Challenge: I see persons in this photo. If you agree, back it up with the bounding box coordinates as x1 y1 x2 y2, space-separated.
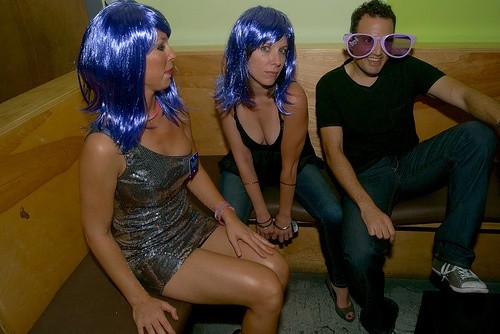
75 1 289 334
212 6 357 323
316 0 500 334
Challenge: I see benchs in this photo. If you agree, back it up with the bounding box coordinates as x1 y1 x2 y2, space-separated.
0 45 500 334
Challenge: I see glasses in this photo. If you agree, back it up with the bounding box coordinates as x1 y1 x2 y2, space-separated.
343 33 416 58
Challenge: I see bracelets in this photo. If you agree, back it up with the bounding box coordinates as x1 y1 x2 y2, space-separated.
280 182 296 187
256 216 273 228
214 200 236 225
243 181 258 185
274 220 291 230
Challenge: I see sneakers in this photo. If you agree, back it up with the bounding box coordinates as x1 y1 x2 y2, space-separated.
431 255 489 294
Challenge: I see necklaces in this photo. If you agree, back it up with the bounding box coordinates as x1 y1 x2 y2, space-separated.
142 101 161 123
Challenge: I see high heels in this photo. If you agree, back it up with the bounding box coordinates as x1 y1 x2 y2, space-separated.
325 272 356 323
